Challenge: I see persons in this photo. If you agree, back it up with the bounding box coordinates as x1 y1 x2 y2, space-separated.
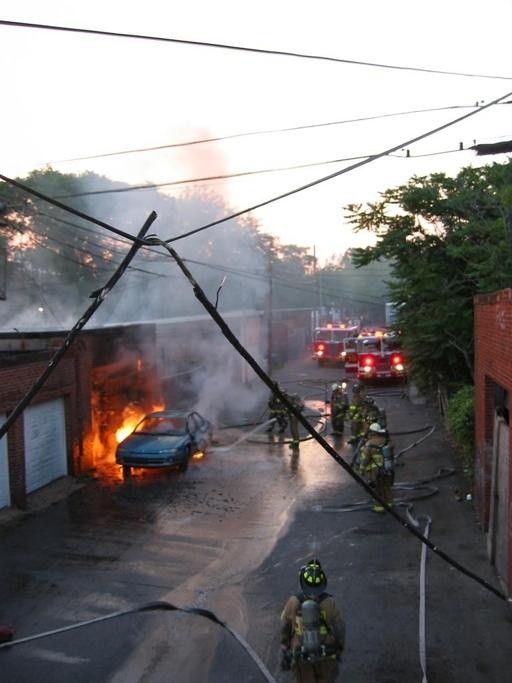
281 391 304 449
327 382 397 513
265 381 288 433
278 558 345 682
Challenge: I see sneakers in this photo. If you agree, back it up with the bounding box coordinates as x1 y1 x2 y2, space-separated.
330 431 342 435
266 427 284 433
371 503 392 513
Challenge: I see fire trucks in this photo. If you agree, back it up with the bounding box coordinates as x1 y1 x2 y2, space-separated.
313 320 409 384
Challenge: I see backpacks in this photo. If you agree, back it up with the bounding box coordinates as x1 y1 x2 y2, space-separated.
291 392 304 411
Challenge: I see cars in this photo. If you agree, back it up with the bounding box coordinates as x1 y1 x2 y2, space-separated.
114 409 214 472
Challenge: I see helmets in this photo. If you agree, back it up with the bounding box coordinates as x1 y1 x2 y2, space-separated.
299 560 326 594
332 383 341 391
370 423 386 434
